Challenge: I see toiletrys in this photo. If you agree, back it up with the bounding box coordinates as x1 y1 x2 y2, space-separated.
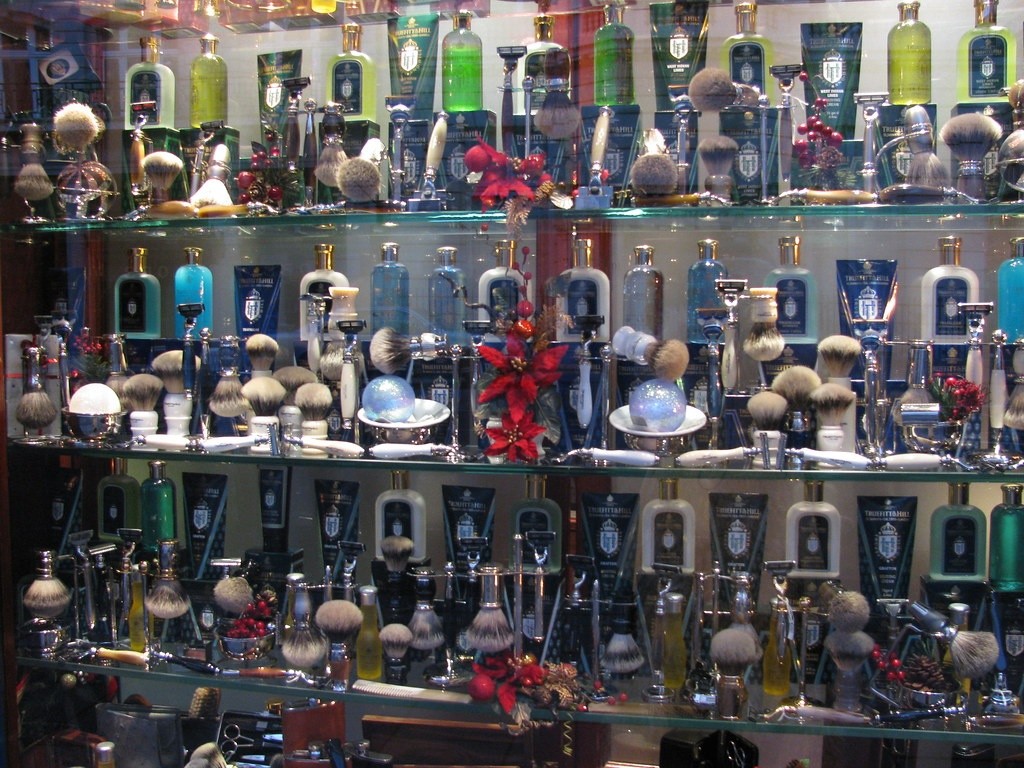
1 0 1024 685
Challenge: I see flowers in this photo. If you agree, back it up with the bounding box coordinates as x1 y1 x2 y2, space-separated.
469 651 628 736
237 107 301 210
929 372 992 457
476 247 572 462
464 134 555 228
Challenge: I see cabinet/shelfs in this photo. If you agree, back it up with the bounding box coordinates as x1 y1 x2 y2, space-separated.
1 1 1024 767
